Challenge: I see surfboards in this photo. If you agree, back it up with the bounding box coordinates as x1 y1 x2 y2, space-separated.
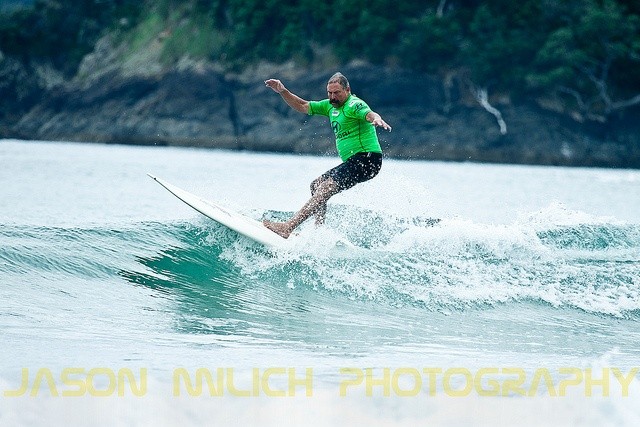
146 172 287 248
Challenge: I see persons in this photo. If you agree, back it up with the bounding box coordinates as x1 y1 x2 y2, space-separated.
261 70 392 240
